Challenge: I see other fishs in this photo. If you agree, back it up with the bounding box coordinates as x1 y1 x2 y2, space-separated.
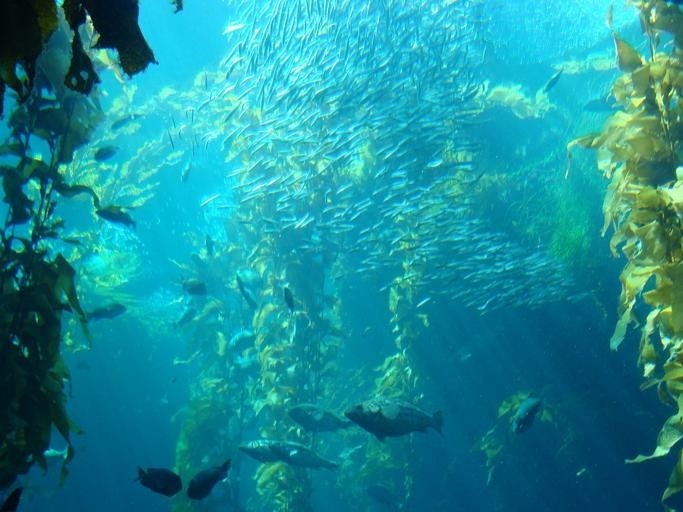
169 0 599 353
